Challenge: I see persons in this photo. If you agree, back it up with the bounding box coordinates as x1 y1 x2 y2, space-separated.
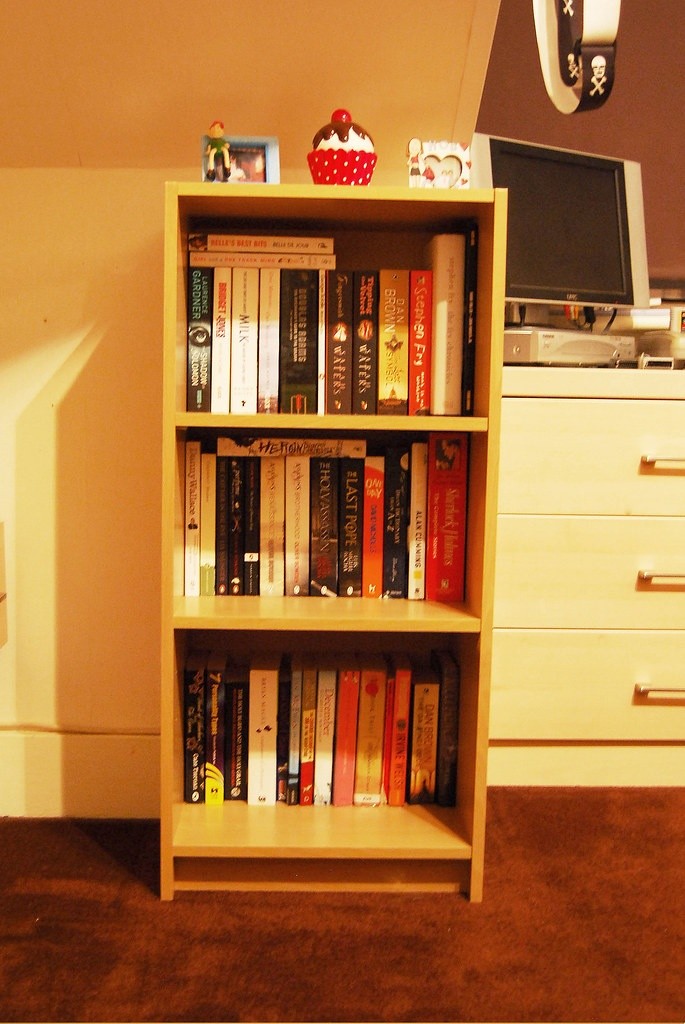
203 119 232 181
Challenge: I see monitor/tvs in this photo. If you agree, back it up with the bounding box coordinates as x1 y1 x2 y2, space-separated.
470 132 650 332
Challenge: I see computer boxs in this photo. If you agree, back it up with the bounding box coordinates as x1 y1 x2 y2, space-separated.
503 330 638 369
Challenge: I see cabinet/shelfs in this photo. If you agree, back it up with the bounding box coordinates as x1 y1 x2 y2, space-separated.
485 366 685 787
160 180 510 905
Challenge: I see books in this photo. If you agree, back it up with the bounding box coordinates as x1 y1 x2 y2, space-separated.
182 649 361 805
376 221 479 415
355 647 460 806
185 437 384 599
385 432 469 602
191 265 377 415
187 231 335 269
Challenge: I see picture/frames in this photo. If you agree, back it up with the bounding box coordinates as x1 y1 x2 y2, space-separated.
405 137 471 189
201 133 282 184
